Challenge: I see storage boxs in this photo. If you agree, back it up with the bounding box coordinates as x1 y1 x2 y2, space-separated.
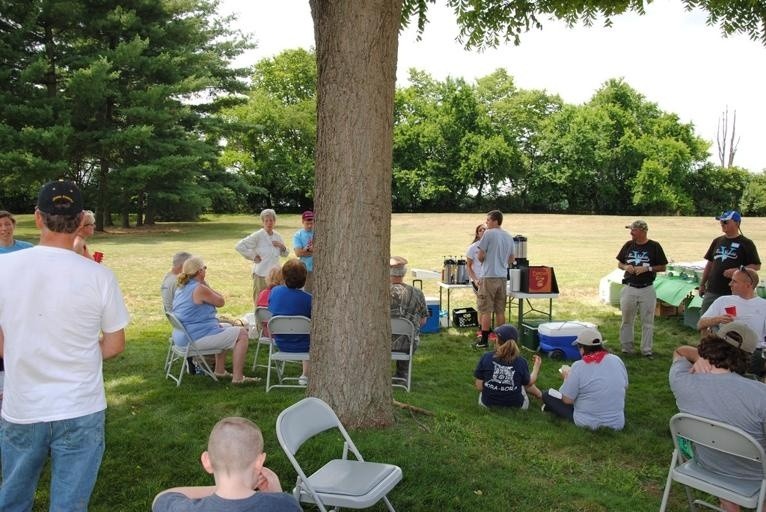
666 265 704 282
600 279 623 304
522 319 546 350
655 297 701 329
520 266 559 293
419 297 439 333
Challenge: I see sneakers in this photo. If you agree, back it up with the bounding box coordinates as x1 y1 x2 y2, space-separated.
476 342 489 350
231 376 261 384
213 371 232 377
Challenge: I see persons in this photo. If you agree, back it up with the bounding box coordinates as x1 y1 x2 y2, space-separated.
73 209 104 264
0 181 130 512
699 211 761 337
151 417 302 512
388 255 430 386
540 327 629 431
669 321 766 512
161 209 315 385
697 264 766 382
0 210 33 254
616 220 668 358
474 324 544 411
466 210 516 348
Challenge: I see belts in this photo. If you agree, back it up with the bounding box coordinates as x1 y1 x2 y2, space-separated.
627 283 652 288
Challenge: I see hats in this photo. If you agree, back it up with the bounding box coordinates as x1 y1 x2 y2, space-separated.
390 256 408 266
572 329 602 345
625 221 648 231
716 210 741 223
303 210 314 221
39 179 83 213
495 325 518 341
717 321 759 354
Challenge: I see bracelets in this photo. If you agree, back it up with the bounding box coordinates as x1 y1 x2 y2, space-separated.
648 266 652 271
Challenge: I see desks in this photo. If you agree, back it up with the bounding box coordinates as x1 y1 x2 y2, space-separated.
439 282 513 329
490 292 558 333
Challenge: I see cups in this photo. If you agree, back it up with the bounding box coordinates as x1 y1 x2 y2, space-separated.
725 304 736 317
93 250 103 262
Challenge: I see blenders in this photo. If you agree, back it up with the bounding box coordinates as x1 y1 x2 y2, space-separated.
507 235 529 293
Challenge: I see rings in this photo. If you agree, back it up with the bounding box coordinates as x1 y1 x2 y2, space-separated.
637 270 639 273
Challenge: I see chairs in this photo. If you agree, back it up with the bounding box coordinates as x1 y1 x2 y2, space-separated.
391 318 414 392
660 413 765 512
265 315 311 392
165 313 219 387
275 396 404 512
252 308 273 372
161 304 174 373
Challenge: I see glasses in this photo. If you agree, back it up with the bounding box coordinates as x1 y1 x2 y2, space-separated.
738 265 753 285
722 221 728 223
